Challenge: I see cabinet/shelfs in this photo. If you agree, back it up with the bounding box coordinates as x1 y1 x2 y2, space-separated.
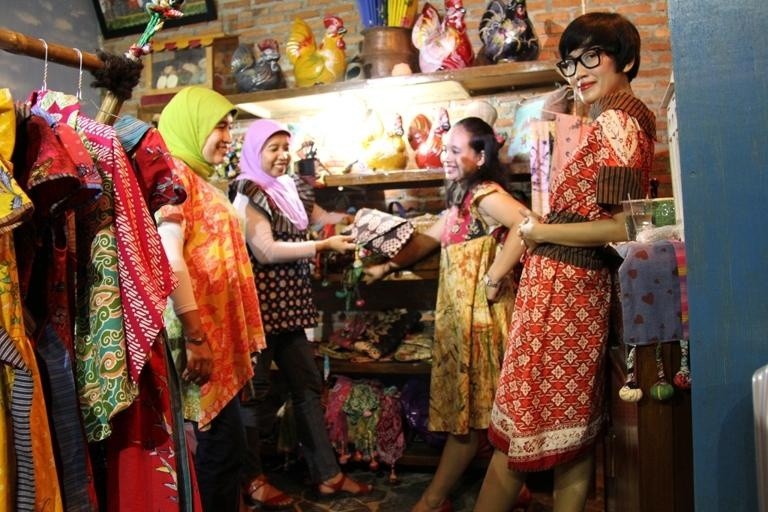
137 59 585 467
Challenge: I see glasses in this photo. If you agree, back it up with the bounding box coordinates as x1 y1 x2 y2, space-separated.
556 46 608 77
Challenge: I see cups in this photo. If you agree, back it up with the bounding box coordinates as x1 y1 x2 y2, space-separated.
621 199 653 241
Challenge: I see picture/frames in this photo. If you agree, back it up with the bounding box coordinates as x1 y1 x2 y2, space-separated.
93 0 217 40
146 36 213 95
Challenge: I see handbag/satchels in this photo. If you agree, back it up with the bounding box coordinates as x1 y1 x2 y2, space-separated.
354 312 421 362
320 311 374 359
394 321 437 362
342 207 413 260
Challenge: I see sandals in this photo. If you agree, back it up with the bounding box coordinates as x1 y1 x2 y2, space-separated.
317 474 372 501
243 478 294 509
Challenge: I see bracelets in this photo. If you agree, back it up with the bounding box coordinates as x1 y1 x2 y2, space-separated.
184 333 211 345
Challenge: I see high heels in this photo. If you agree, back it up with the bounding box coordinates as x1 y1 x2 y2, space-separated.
439 498 454 512
511 485 532 512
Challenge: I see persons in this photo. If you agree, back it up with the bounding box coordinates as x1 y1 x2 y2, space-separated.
236 119 373 509
155 86 266 510
472 13 656 511
363 117 531 512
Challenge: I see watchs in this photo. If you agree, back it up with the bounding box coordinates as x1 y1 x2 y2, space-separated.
482 275 500 288
